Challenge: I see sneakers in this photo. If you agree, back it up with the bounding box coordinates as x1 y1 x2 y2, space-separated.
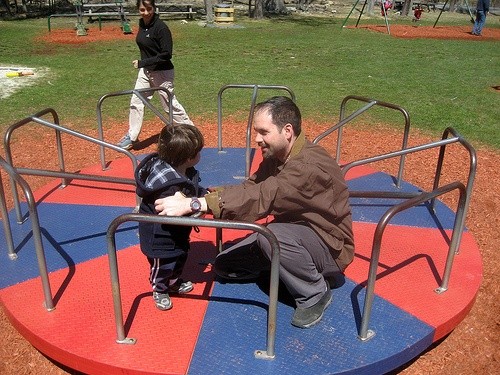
116 135 141 151
291 281 333 329
176 281 193 296
153 291 172 311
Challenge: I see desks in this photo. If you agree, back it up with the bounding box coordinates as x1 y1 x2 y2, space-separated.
82 4 127 22
155 3 193 20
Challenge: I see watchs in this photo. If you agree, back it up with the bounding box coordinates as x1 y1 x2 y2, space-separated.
190 197 201 213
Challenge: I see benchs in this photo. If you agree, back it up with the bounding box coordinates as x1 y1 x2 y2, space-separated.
155 11 196 15
83 12 129 16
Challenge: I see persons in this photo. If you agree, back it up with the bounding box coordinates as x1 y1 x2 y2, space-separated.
471 0 490 35
134 123 212 311
154 96 355 327
427 0 436 12
114 0 194 149
381 0 392 16
